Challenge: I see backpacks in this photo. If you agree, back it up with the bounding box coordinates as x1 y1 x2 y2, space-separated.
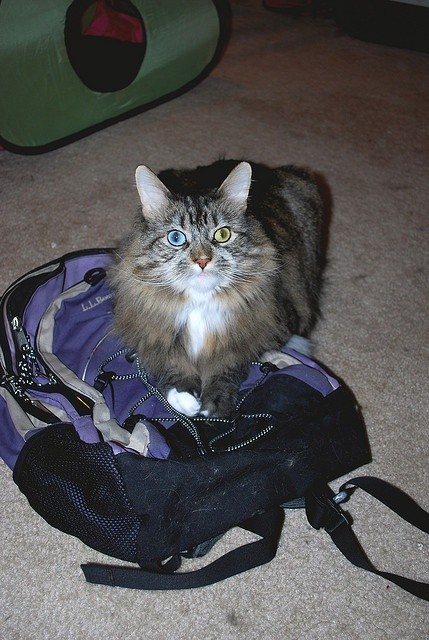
2 248 429 600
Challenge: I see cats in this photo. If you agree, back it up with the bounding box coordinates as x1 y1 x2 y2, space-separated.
103 153 334 419
